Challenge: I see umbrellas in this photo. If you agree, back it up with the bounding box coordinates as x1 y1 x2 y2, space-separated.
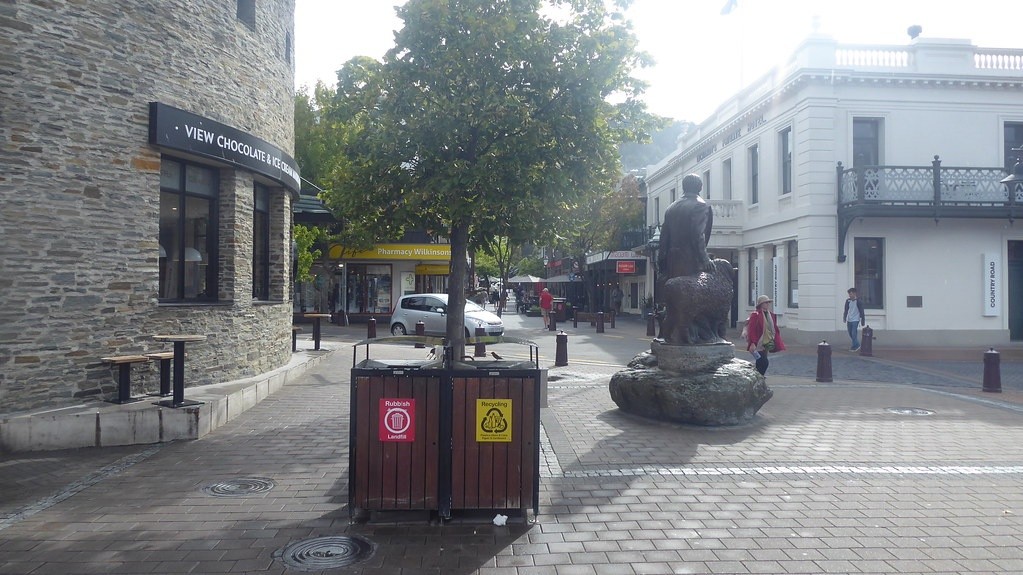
478 273 582 297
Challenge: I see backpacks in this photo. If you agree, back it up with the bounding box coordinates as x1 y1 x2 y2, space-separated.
740 312 759 341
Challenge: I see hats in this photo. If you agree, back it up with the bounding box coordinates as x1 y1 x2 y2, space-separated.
756 295 773 306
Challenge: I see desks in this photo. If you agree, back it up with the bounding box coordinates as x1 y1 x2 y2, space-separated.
304 313 332 351
152 336 207 408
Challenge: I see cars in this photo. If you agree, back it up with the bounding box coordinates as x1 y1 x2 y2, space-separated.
390 293 506 346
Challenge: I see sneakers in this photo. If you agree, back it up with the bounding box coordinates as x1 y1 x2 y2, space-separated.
853 345 861 351
849 346 853 351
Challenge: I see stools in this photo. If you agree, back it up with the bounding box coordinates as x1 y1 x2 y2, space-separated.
143 352 188 397
101 355 149 404
292 327 302 352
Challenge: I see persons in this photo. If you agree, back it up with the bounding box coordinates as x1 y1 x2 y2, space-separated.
747 296 785 378
612 286 623 316
493 288 499 306
501 289 508 311
659 173 718 278
480 288 488 310
842 288 865 351
429 300 441 312
539 288 553 330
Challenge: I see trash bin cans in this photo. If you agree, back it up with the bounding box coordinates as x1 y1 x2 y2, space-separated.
447 336 541 517
349 336 447 520
556 302 566 322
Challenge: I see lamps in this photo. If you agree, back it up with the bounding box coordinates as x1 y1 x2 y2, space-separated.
197 251 211 265
158 244 165 258
173 246 202 262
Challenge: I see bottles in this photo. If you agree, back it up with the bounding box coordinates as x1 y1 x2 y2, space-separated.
752 350 761 360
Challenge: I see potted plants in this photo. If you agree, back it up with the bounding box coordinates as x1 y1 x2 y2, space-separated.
526 305 541 317
